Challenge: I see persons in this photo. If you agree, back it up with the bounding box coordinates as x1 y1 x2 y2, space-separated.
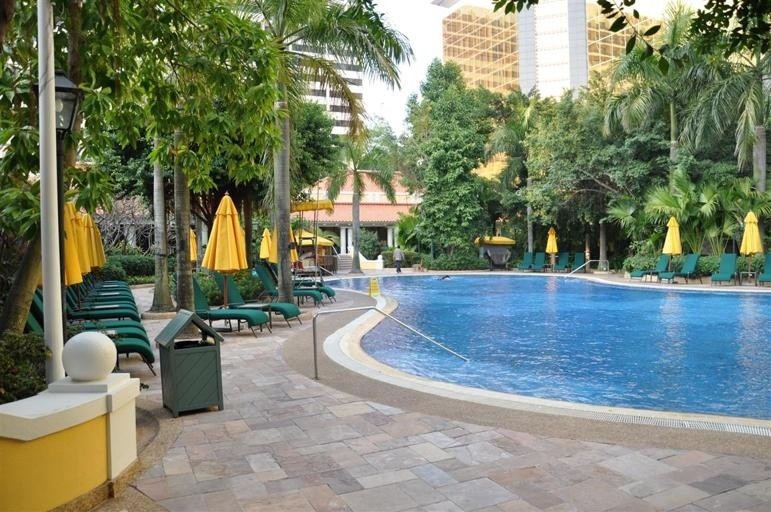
393 245 405 273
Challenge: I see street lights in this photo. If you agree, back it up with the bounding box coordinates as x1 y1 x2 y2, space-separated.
33 70 85 377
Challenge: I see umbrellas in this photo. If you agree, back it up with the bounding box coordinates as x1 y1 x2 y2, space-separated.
35 199 83 288
190 229 198 265
546 226 559 265
259 228 273 261
739 209 764 281
268 223 299 265
663 215 683 270
73 210 91 308
91 220 107 288
201 190 249 308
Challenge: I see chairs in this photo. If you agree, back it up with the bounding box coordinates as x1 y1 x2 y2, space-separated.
254 262 337 307
517 250 585 274
170 271 272 339
213 273 302 329
629 252 771 286
26 264 155 377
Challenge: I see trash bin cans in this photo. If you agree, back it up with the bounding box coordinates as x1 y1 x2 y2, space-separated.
318 256 337 275
155 309 225 418
